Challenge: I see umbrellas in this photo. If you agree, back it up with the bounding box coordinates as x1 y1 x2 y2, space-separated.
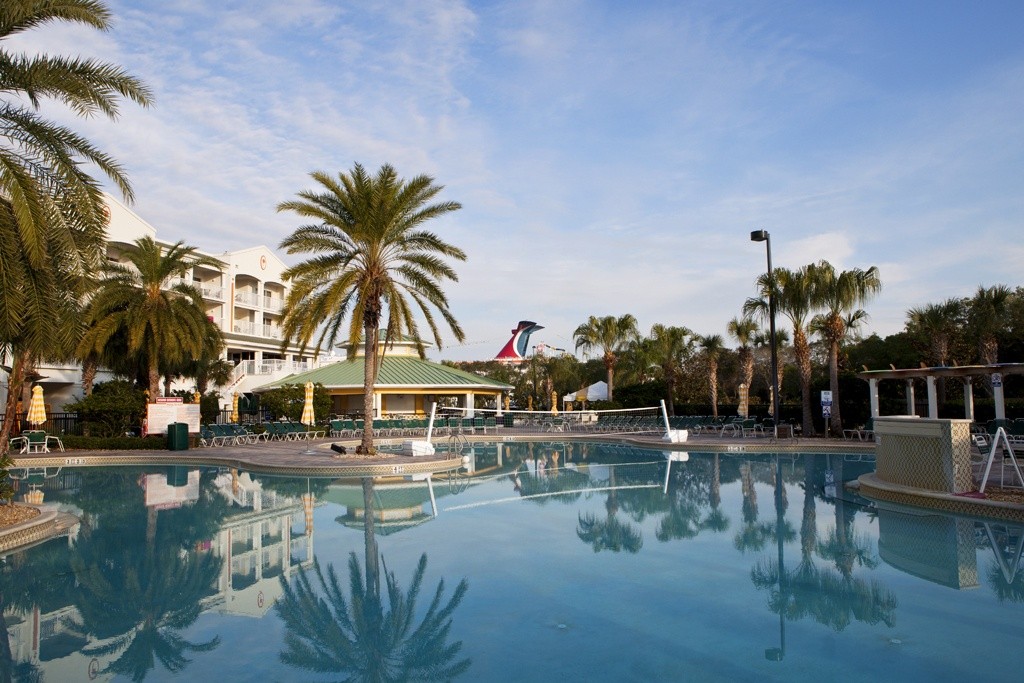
504 390 574 411
229 392 240 423
301 381 316 451
737 383 749 417
772 463 778 494
769 386 774 416
299 477 317 536
26 384 47 429
23 483 45 505
230 468 239 497
738 464 753 502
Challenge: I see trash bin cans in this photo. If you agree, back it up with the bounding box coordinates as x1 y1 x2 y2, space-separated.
167 466 188 487
168 422 189 451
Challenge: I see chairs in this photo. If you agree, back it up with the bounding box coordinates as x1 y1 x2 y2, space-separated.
42 428 66 453
973 435 1006 484
1001 439 1024 492
6 430 27 454
199 415 875 449
23 430 47 455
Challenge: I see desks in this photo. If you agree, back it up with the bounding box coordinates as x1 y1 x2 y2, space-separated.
20 432 51 455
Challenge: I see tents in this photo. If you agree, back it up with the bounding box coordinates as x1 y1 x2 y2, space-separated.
563 453 612 483
562 380 607 418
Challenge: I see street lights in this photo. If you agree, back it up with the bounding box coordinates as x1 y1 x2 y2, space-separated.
750 228 782 439
